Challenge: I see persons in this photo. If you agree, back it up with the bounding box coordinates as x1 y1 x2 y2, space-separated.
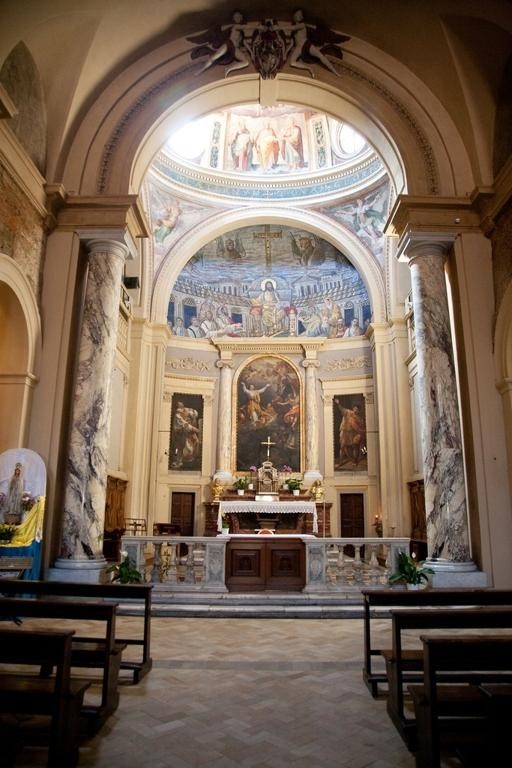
224 238 243 259
171 281 367 469
289 232 325 267
254 119 278 168
231 118 253 170
281 116 303 169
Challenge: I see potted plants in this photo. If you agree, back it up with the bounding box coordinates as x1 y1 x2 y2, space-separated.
285 477 303 495
385 549 437 589
233 478 247 495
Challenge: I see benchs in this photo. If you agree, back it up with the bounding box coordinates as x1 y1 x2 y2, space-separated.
0 574 156 768
361 585 512 767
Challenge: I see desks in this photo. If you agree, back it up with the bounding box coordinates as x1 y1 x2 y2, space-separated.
218 501 317 536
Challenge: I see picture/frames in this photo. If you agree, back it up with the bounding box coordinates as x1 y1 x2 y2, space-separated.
228 350 308 476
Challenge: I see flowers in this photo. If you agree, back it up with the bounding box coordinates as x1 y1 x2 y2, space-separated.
279 463 292 482
248 465 256 483
0 520 20 539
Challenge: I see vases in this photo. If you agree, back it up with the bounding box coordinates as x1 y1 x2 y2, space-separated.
283 483 288 488
0 539 11 544
247 483 253 489
